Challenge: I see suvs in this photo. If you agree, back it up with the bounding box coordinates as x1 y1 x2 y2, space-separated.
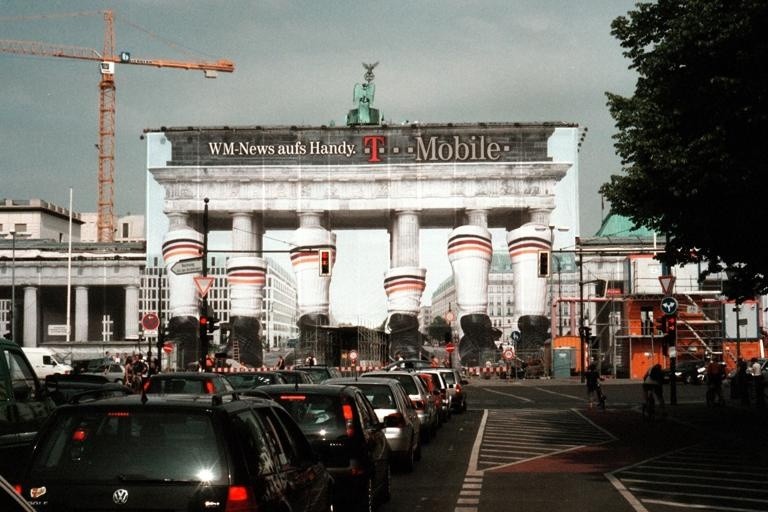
13 380 337 512
0 338 59 510
364 368 466 447
319 375 421 473
246 377 401 512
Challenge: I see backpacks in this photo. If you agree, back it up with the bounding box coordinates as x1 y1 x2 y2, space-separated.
649 364 662 382
707 364 723 382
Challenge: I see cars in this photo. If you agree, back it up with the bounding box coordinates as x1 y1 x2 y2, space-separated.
39 359 344 406
660 359 725 387
723 356 767 392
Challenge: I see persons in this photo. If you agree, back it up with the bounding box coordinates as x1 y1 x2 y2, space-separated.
102 347 164 394
390 350 405 371
431 354 440 367
641 362 669 416
704 356 728 406
276 355 285 370
749 357 764 402
580 363 607 410
735 357 747 397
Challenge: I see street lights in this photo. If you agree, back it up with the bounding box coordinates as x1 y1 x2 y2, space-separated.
9 229 18 342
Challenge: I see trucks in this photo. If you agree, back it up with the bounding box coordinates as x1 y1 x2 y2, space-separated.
4 346 74 377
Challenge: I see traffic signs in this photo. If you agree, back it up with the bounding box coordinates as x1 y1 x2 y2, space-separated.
171 258 203 275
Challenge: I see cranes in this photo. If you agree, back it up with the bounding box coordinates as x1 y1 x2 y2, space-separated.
0 10 235 242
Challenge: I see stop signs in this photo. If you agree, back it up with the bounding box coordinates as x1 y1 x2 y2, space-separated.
164 343 172 353
445 344 456 353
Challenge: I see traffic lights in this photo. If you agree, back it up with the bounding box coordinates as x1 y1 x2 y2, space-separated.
199 316 207 341
666 315 676 335
655 316 665 334
208 317 219 333
319 249 330 277
537 250 549 278
584 327 592 343
